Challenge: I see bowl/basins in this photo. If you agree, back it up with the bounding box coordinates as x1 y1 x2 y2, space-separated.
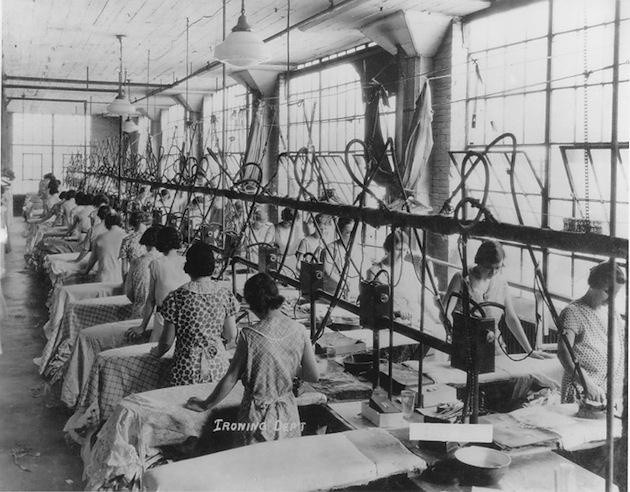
342 352 374 376
454 445 511 487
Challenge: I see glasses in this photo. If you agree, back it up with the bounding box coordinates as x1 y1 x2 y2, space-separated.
481 261 507 271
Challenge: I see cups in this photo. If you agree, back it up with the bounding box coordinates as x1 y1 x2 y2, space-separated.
402 392 415 418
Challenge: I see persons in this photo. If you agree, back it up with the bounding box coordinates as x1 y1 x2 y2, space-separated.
186 272 326 448
38 164 367 303
120 227 163 319
152 239 239 390
124 226 188 346
365 229 440 326
556 259 626 404
439 241 554 360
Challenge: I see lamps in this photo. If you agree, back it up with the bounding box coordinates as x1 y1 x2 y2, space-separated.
104 1 273 137
